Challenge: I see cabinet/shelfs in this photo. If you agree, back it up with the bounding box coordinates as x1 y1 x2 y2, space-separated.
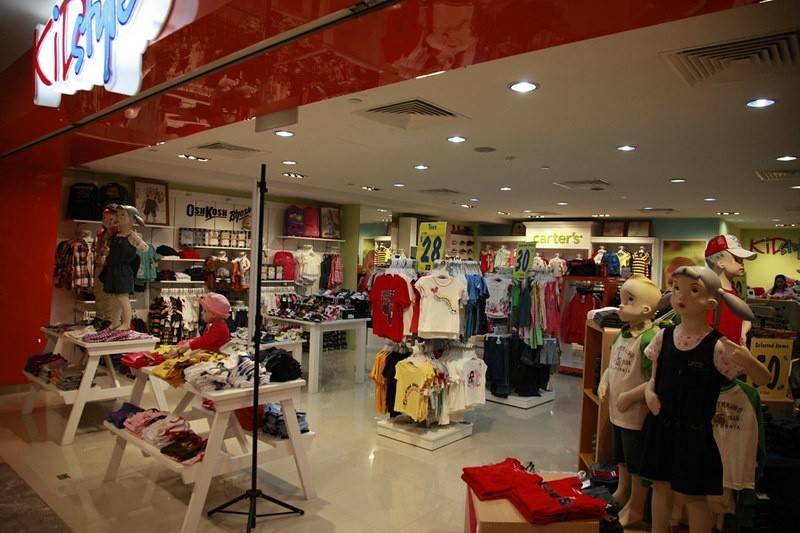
22 319 169 446
468 480 600 532
157 257 205 284
578 319 637 485
101 357 313 533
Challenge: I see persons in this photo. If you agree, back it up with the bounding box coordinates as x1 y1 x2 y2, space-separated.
592 273 663 529
178 292 233 356
624 262 775 533
85 204 123 328
767 274 796 298
100 203 150 332
698 234 759 355
655 256 697 322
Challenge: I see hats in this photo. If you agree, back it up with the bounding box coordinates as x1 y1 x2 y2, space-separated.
198 292 231 320
704 234 758 262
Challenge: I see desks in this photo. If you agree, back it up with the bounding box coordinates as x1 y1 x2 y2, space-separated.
265 314 371 394
257 332 306 371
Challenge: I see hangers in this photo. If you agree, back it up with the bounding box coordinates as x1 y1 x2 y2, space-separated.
158 287 207 298
492 265 556 284
385 336 478 364
386 256 483 276
485 326 558 345
483 244 649 260
81 230 95 243
